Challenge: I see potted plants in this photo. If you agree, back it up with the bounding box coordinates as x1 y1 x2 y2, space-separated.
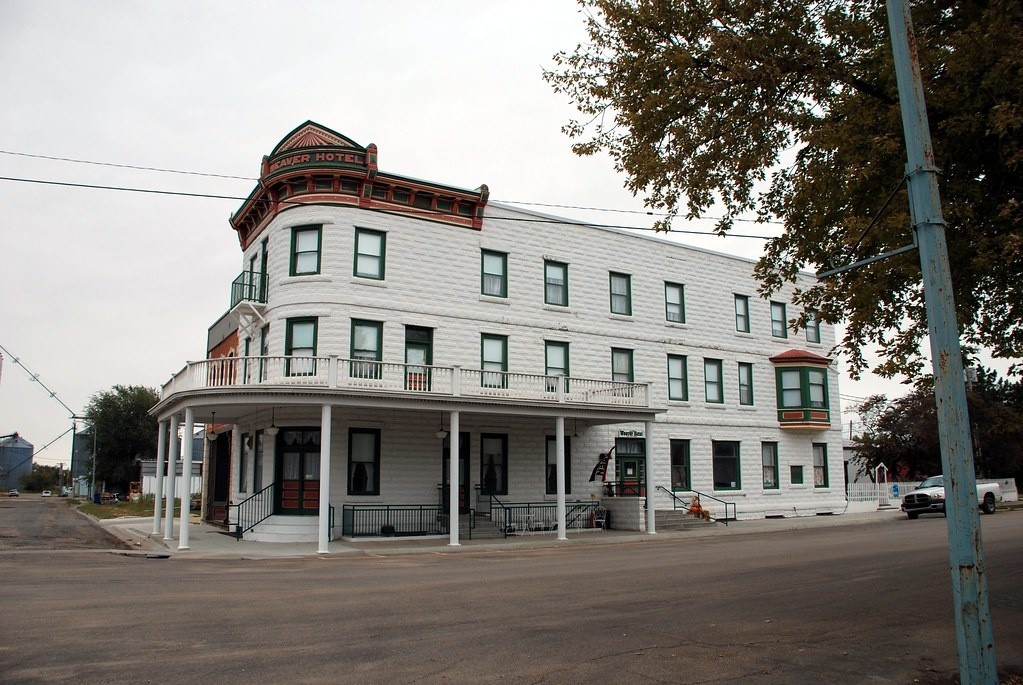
190 492 201 510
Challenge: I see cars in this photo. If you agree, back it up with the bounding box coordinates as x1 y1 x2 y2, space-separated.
8 488 19 497
41 490 52 497
58 491 68 497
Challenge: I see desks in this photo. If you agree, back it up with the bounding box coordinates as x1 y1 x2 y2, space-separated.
518 514 535 539
570 512 587 534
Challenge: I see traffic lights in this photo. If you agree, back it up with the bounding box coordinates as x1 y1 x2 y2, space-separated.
62 470 67 478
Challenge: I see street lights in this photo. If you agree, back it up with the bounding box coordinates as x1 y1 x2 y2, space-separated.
72 420 97 503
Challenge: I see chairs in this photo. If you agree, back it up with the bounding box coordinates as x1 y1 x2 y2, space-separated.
548 508 558 536
592 506 607 536
529 508 545 538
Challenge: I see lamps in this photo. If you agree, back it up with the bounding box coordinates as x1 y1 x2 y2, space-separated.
266 405 280 435
572 418 582 445
436 410 448 438
206 412 219 441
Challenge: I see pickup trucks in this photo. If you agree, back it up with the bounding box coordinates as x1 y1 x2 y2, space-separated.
900 474 1002 520
